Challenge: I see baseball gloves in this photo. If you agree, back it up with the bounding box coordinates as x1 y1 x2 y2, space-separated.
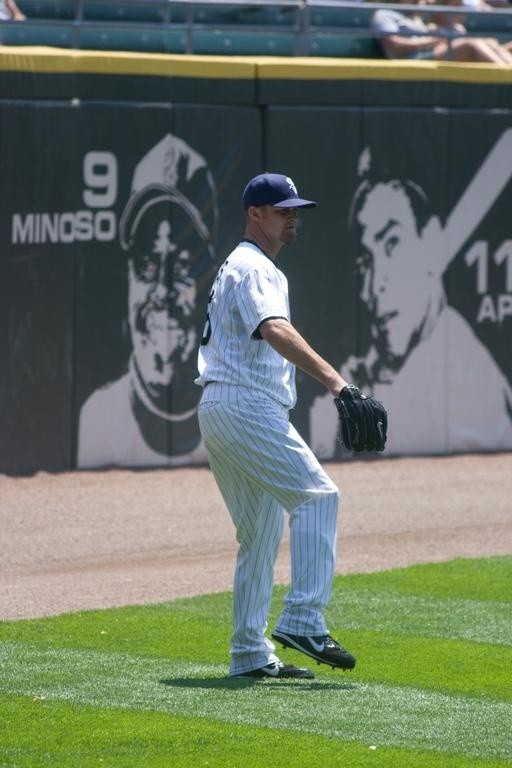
333 384 388 452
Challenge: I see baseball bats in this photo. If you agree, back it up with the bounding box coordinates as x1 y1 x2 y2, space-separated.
363 130 511 373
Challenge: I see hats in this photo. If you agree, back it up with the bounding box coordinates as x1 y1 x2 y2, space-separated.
242 171 317 210
347 141 439 221
118 131 220 261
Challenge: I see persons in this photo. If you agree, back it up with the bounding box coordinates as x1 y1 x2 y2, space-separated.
366 0 512 63
192 171 389 680
74 130 228 472
427 0 512 54
306 158 512 456
0 0 31 23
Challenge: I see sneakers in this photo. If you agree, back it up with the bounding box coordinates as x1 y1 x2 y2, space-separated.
230 661 315 680
271 629 356 672
